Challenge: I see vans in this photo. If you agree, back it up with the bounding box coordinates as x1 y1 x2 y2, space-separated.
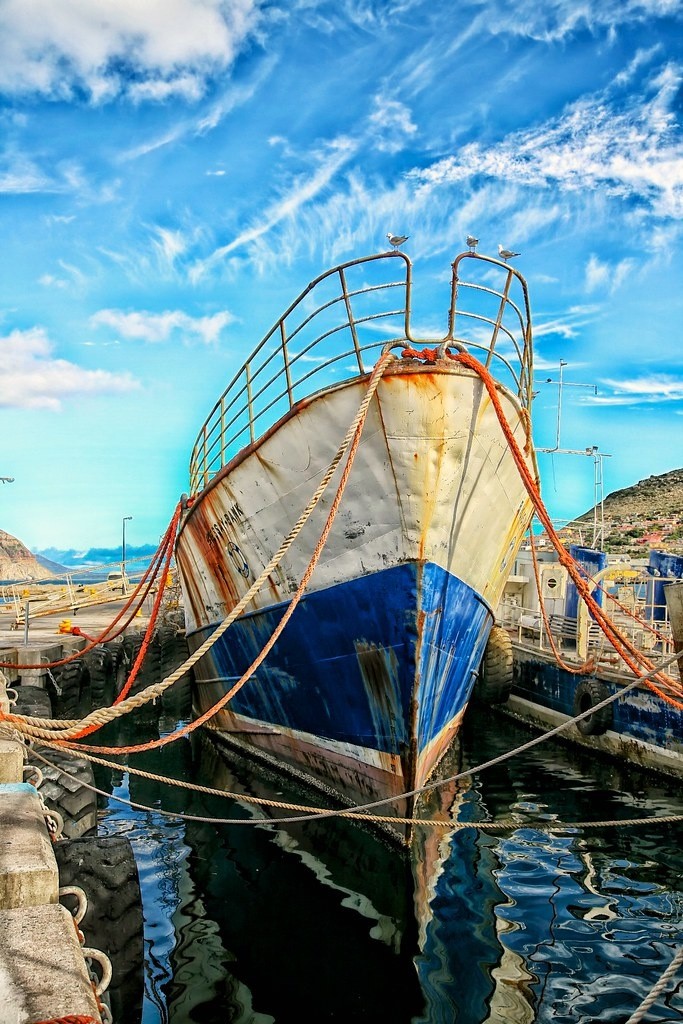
107 571 131 591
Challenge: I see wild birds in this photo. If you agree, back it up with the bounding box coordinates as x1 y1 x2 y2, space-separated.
386 232 410 252
497 244 521 264
466 235 479 254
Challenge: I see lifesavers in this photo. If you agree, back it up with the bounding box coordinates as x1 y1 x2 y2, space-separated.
50 622 196 744
4 685 53 757
474 623 513 706
573 679 612 738
23 742 100 837
51 833 144 1024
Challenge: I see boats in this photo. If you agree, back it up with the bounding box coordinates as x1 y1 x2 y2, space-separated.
172 247 544 848
488 515 683 781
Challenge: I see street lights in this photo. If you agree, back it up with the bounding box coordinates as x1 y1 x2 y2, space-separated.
120 517 134 596
